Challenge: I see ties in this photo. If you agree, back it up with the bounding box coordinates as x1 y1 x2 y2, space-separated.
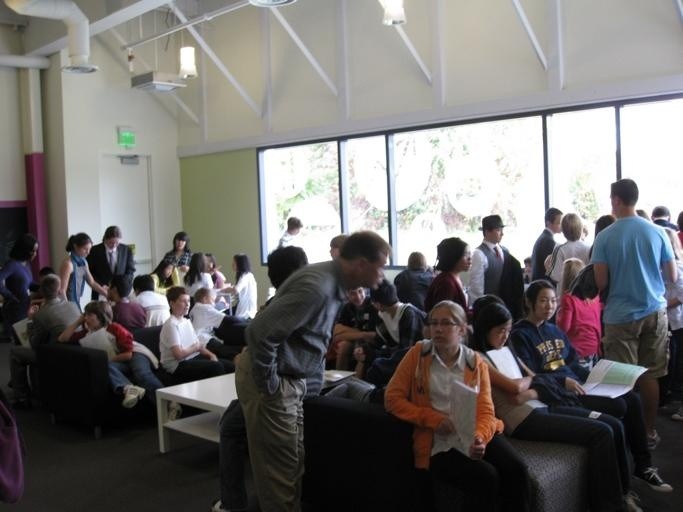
493 247 502 259
108 251 116 273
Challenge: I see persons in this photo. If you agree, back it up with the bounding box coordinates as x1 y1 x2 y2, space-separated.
159 286 228 380
472 300 645 512
466 214 513 324
191 287 247 361
529 208 562 288
509 280 673 512
219 254 260 322
426 237 473 337
57 302 182 439
209 245 310 512
329 232 348 263
555 259 603 357
4 273 87 411
0 234 40 299
277 218 302 249
637 207 683 423
132 272 172 327
183 252 225 307
592 179 679 453
649 205 672 230
156 230 195 286
353 277 428 384
546 216 591 298
86 226 136 304
107 272 150 330
59 232 111 319
37 263 56 293
0 274 30 343
588 214 615 259
679 209 683 245
151 260 179 292
396 251 437 310
384 300 533 511
332 280 381 379
524 257 536 292
204 253 228 291
235 231 390 512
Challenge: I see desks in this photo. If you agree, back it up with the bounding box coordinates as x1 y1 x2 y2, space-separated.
155 370 359 453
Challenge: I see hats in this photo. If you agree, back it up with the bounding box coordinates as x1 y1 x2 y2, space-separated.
478 215 506 230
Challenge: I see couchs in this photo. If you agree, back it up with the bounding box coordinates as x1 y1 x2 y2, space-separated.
303 397 595 512
27 316 248 430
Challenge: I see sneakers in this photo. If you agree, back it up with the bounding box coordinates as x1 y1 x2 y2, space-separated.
647 432 661 451
123 386 145 407
632 468 673 492
169 403 182 422
625 492 643 512
672 406 683 421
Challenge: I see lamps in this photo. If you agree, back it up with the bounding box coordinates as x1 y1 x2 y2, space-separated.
179 47 197 79
378 1 406 25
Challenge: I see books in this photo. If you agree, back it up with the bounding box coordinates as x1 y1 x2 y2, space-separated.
577 359 647 398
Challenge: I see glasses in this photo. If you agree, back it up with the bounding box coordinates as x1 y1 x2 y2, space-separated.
430 322 461 329
